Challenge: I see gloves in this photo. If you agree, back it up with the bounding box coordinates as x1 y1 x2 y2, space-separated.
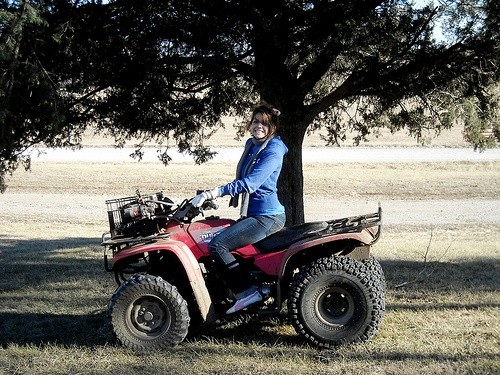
189 186 220 208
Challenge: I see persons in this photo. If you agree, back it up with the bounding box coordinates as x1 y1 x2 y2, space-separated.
192 103 288 314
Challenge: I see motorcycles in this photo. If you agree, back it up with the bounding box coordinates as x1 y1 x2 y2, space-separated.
102 191 387 353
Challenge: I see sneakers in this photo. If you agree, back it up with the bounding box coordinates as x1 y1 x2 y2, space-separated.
226 290 263 314
